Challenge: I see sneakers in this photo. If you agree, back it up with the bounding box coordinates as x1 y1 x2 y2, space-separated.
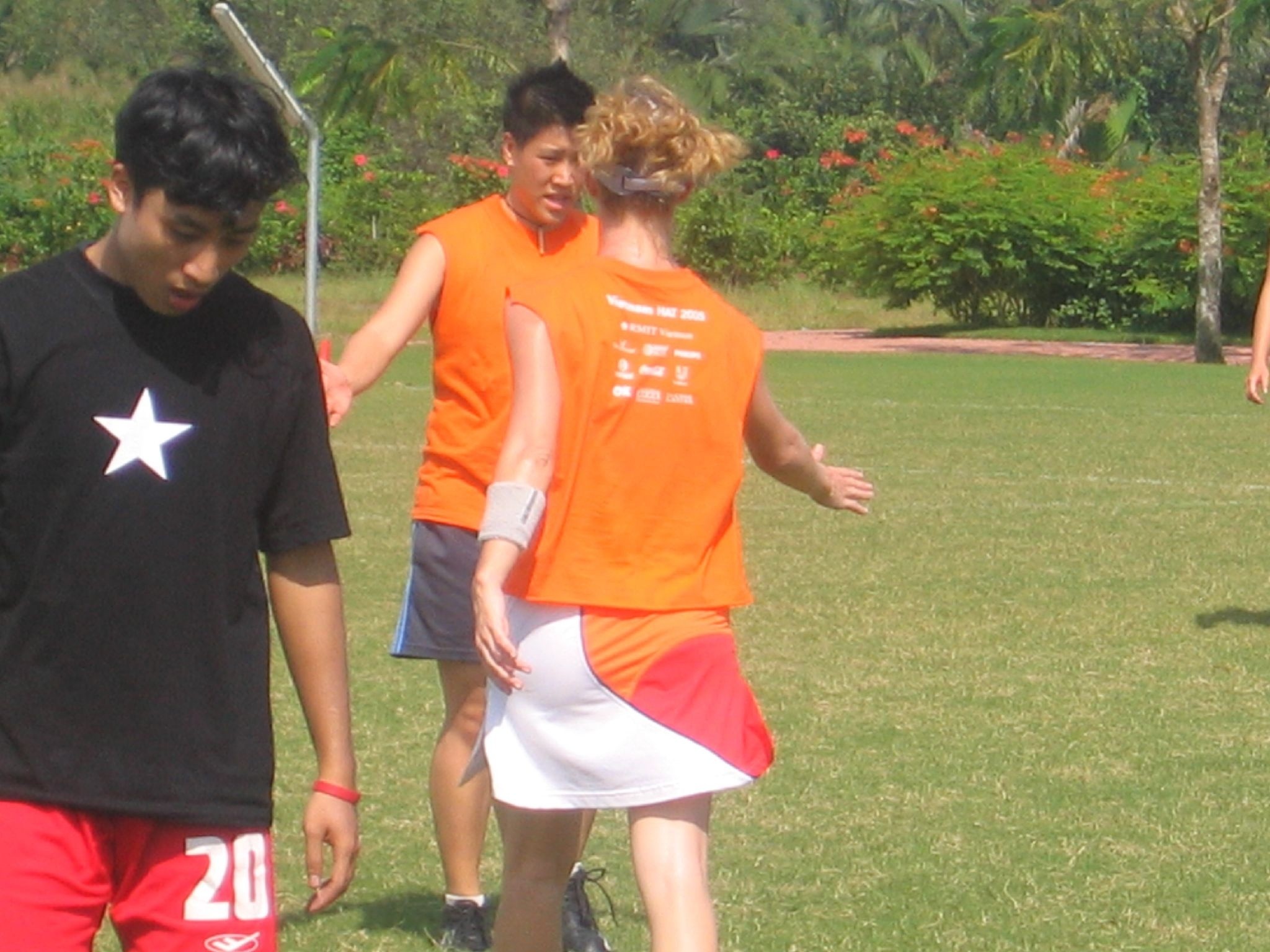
443 891 490 952
562 861 609 952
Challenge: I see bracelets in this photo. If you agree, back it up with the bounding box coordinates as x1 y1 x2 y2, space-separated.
312 780 361 804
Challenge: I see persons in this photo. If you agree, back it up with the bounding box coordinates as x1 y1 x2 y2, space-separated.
0 67 364 952
1245 258 1270 405
472 76 876 952
318 59 616 952
